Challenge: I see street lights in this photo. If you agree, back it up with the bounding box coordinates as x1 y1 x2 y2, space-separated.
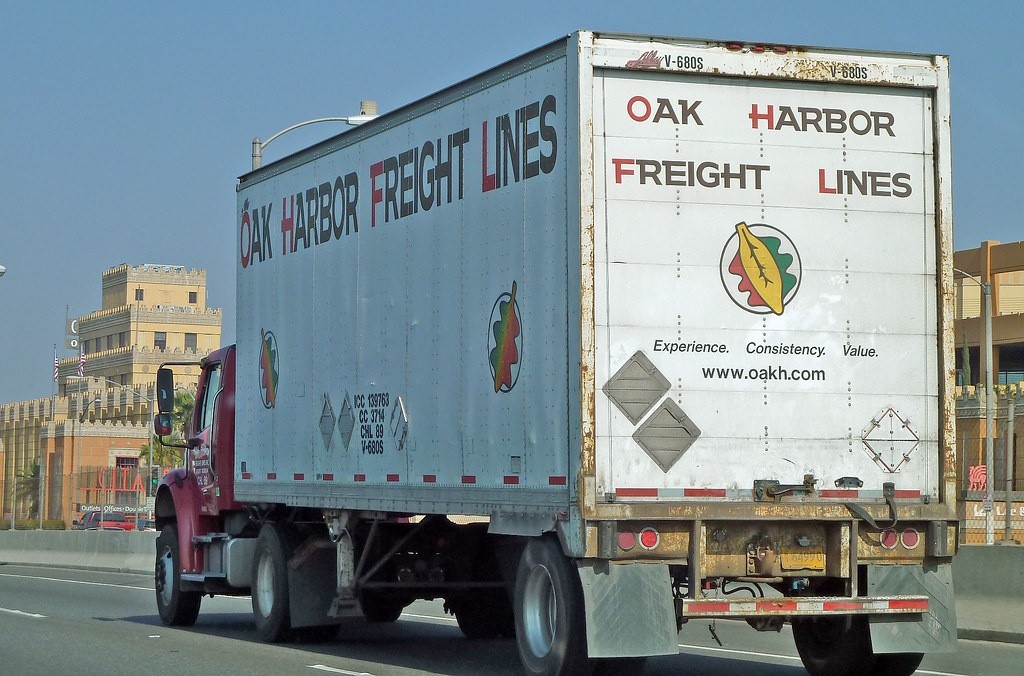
66 376 152 519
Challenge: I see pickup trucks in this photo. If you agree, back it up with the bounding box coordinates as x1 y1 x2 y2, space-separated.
71 510 136 531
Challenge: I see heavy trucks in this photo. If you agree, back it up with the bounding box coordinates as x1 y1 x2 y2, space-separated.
152 29 959 676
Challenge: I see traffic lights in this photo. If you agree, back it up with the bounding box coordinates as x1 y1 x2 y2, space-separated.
151 465 160 489
